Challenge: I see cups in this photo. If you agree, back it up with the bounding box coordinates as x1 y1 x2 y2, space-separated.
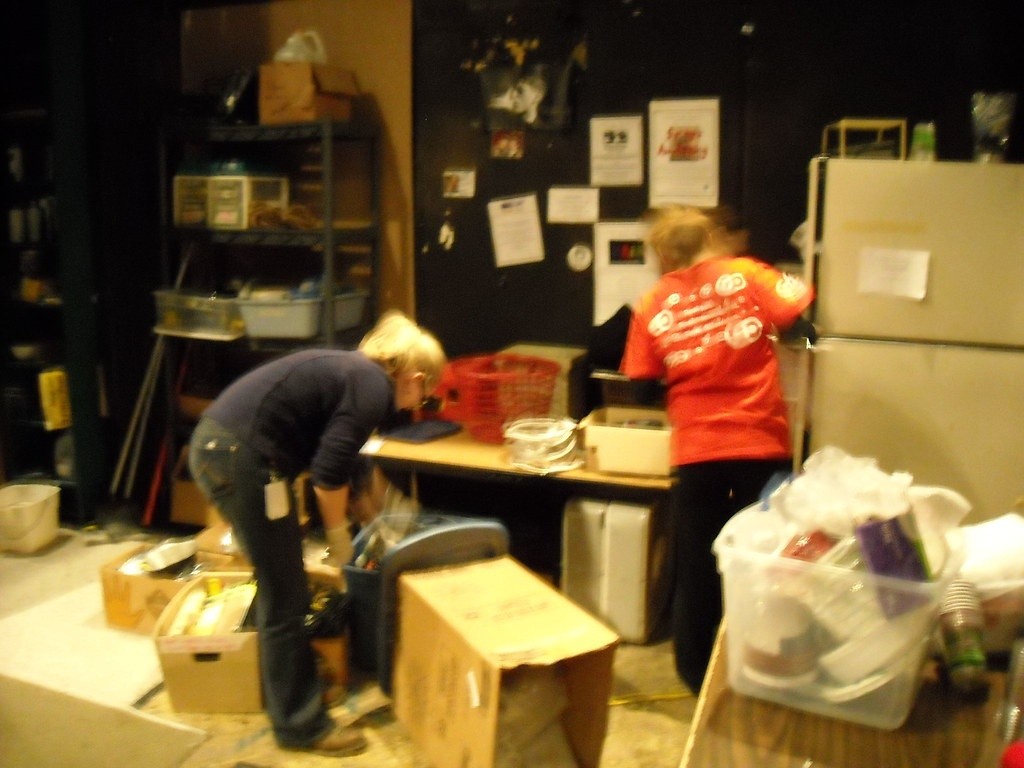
938 577 989 690
995 639 1024 743
907 120 938 159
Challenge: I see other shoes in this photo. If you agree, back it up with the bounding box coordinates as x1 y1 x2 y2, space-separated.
281 726 368 756
324 686 347 707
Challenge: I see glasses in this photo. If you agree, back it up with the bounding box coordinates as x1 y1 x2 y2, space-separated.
418 380 428 407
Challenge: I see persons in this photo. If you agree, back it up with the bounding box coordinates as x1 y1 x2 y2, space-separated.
188 315 445 757
618 206 823 697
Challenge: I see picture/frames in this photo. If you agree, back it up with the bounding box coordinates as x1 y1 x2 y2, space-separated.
546 184 601 228
485 190 548 273
591 217 663 330
645 95 725 212
489 126 530 165
443 167 478 201
587 112 648 189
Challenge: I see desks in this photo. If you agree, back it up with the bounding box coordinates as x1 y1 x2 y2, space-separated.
357 421 683 511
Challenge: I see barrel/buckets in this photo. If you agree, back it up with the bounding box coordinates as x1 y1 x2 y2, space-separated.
0 484 62 555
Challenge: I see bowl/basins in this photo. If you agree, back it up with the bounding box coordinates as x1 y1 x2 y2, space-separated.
145 540 200 576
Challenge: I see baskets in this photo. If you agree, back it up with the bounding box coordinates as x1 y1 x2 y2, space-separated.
442 354 563 444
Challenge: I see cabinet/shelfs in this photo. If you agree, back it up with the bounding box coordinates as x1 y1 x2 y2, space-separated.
151 106 381 361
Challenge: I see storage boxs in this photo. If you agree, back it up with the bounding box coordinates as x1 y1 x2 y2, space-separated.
575 405 675 479
151 287 369 341
258 62 361 127
174 173 292 233
101 444 967 768
